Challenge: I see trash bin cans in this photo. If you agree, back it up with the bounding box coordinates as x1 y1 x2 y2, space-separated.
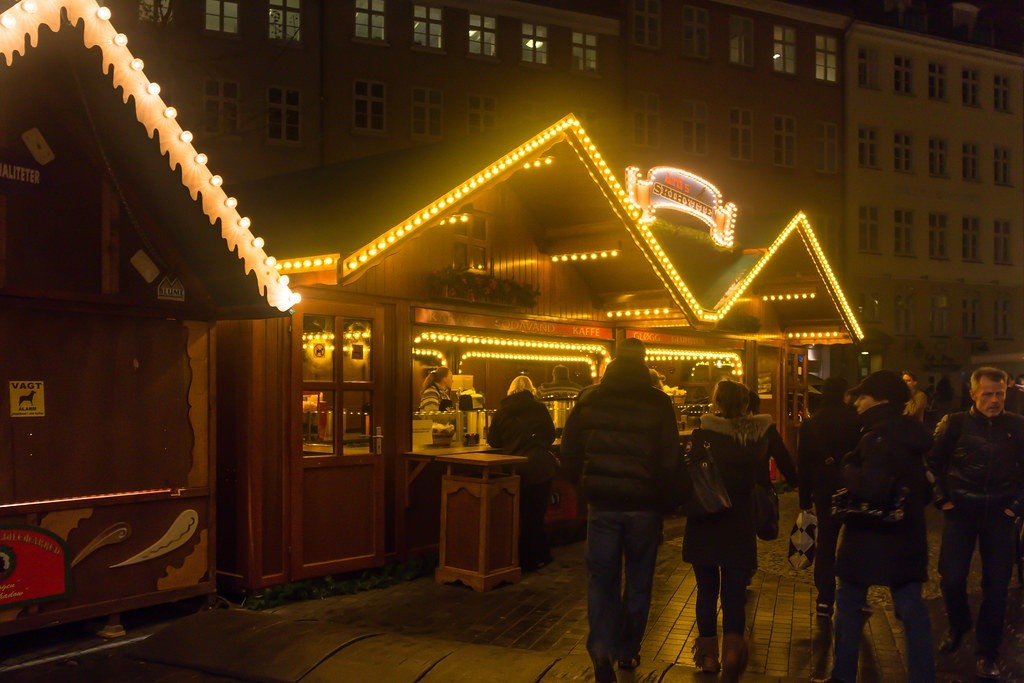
434 452 528 593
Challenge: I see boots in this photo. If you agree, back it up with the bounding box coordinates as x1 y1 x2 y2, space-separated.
721 631 746 677
691 636 721 673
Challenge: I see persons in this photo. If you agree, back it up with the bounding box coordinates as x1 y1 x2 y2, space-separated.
900 369 927 420
924 366 1024 679
924 374 950 429
746 390 798 491
648 369 666 390
798 376 873 617
1005 373 1024 415
488 376 556 570
827 370 936 683
559 338 680 683
420 367 454 412
551 365 570 382
681 380 773 683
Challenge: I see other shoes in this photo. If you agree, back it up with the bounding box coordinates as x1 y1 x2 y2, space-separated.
589 642 619 683
618 656 640 670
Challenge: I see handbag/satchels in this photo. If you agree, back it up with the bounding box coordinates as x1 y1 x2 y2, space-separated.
688 431 732 514
538 452 562 485
789 510 816 571
753 480 779 541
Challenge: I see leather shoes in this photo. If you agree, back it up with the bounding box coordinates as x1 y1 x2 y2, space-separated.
937 616 973 654
976 656 1001 680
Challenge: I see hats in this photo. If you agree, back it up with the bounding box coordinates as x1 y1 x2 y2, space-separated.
847 369 912 404
